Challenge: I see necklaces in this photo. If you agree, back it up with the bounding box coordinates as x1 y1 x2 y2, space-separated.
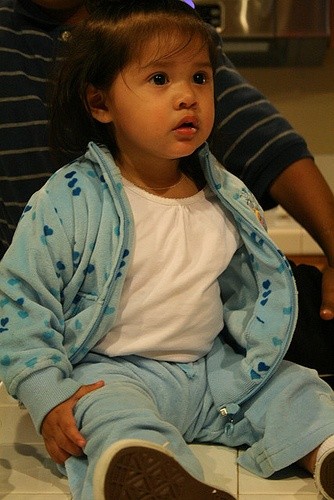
125 172 189 194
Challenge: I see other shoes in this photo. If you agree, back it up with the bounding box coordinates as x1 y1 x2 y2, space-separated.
314 447 334 500
93 439 238 500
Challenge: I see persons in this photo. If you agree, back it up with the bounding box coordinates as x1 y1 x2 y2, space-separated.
0 0 334 500
1 0 334 390
